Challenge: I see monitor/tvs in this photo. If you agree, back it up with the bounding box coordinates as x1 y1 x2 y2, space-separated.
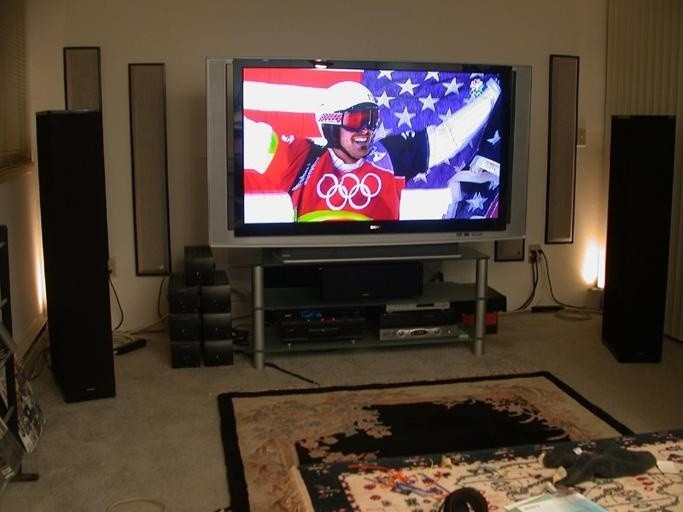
232 58 512 236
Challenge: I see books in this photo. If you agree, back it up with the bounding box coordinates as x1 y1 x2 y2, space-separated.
504 492 610 512
0 352 48 453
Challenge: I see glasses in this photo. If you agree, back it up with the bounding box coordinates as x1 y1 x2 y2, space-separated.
338 109 378 131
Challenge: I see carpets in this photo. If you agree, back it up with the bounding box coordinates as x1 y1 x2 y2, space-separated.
219 370 639 508
295 427 681 508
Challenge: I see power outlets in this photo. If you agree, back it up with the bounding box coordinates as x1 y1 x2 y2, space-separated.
530 246 542 263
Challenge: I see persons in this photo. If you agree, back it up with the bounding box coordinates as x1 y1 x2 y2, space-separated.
243 81 501 223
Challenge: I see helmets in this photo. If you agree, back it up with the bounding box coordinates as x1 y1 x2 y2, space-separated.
316 81 378 136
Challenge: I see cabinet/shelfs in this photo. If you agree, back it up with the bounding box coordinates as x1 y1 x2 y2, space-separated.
231 243 490 371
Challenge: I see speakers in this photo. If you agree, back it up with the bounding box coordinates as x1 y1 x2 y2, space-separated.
65 48 100 112
37 109 116 404
601 115 676 363
131 65 170 274
547 56 578 243
314 260 423 302
